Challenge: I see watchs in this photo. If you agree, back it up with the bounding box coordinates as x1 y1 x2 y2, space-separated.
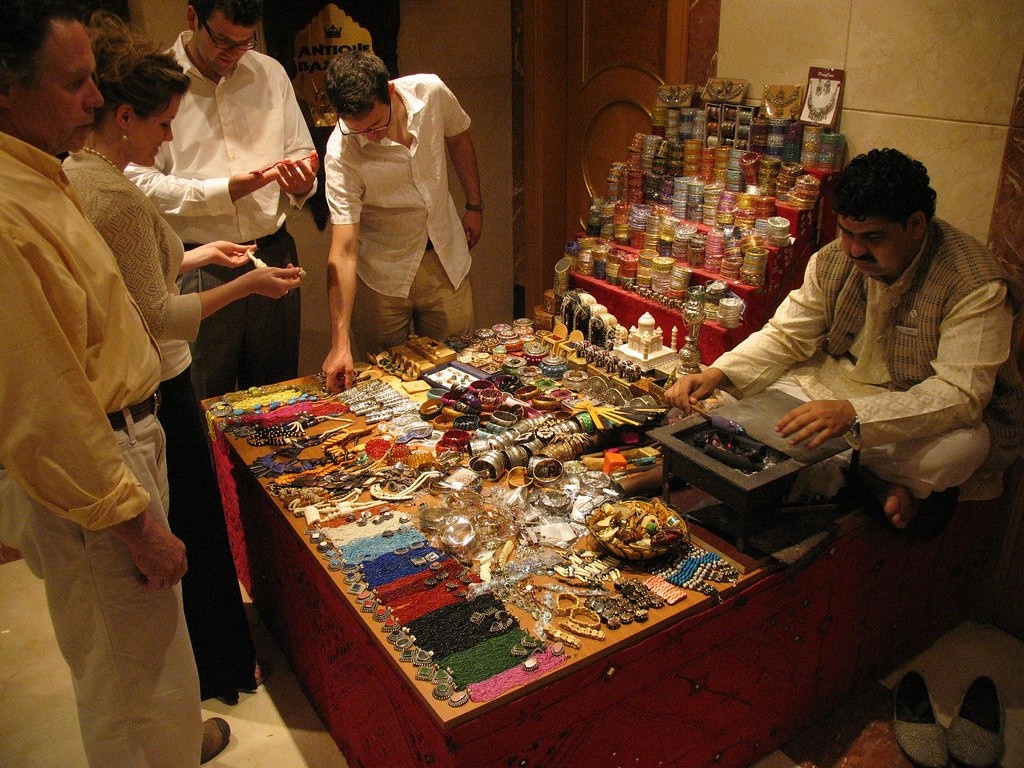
850 416 861 438
465 199 483 213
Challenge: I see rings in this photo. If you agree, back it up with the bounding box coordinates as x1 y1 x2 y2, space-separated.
435 365 473 391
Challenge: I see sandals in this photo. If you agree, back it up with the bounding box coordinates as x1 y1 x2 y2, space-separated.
237 659 264 691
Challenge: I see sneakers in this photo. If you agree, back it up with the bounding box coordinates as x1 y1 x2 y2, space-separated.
200 717 230 764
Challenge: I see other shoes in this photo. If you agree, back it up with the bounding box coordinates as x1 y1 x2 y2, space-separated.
945 675 1004 768
892 671 948 768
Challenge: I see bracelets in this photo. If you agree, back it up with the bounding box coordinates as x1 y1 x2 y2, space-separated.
544 576 664 648
421 412 595 552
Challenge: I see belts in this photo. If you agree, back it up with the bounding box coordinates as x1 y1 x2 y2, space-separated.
106 388 161 431
183 221 286 251
426 238 433 250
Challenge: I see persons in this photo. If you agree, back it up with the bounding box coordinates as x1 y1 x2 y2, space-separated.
664 148 1024 528
138 0 319 404
60 12 307 705
322 51 484 392
0 1 233 768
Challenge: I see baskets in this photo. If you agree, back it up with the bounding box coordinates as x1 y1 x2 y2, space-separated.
585 497 691 563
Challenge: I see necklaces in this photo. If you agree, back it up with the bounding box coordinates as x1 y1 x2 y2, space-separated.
245 414 446 516
83 148 120 174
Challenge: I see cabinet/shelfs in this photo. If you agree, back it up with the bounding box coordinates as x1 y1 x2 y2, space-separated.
535 66 849 367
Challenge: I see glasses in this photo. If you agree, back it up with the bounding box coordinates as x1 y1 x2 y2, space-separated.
337 95 391 136
203 21 258 51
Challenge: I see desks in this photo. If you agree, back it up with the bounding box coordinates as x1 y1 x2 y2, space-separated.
201 362 861 768
